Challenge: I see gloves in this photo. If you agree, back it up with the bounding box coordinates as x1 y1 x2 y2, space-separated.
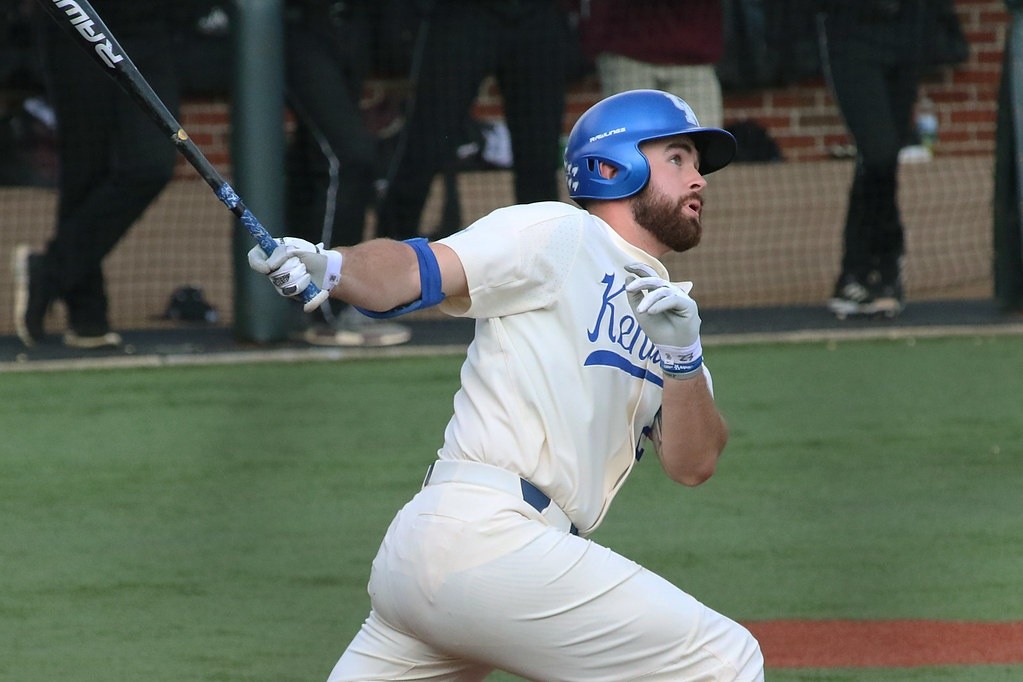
623 262 704 374
248 239 344 312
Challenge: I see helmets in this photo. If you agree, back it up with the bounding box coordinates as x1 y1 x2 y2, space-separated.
564 89 737 207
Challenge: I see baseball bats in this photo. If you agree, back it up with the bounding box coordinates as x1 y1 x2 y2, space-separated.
35 0 333 315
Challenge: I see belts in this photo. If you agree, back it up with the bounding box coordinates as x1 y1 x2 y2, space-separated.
425 465 578 535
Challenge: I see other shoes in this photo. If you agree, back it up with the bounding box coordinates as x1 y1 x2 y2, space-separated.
303 302 408 349
63 320 118 350
14 245 50 348
830 280 906 321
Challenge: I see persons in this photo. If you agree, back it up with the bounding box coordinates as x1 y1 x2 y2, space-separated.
13 0 1023 352
247 88 768 682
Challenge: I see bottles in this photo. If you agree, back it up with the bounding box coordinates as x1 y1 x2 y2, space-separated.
916 99 939 158
